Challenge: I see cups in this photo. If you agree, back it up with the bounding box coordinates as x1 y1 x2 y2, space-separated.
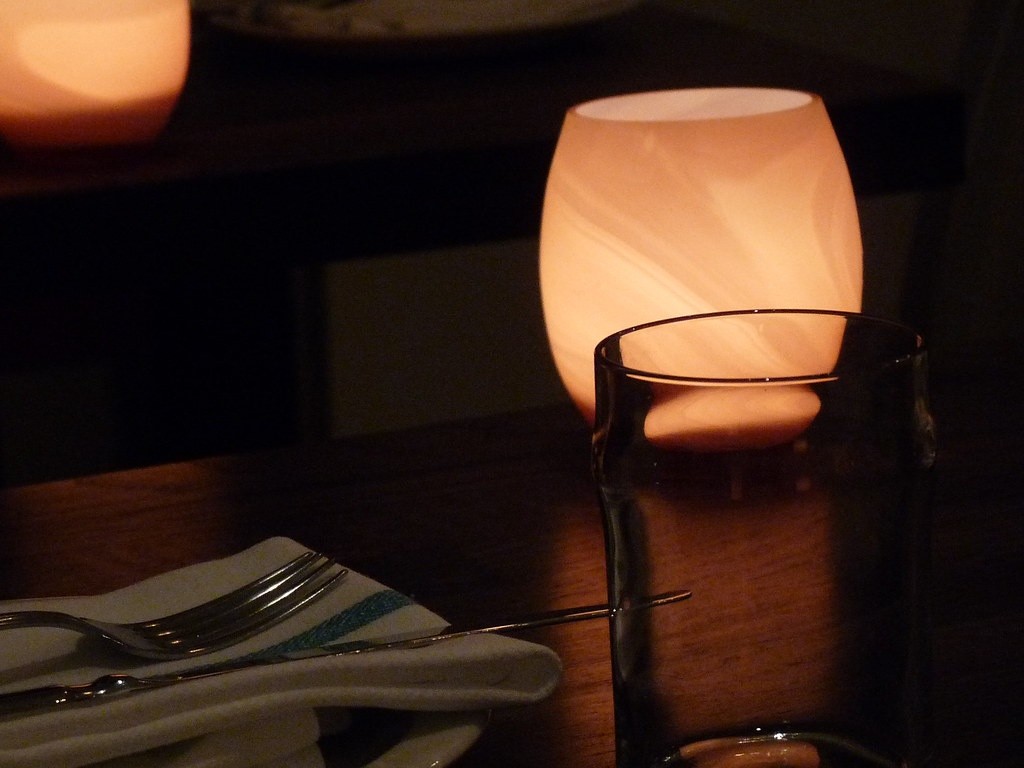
592 310 937 768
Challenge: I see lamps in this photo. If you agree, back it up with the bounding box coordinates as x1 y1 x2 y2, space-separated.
535 83 868 458
0 2 191 148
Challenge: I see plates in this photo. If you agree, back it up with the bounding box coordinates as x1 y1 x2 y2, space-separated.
365 709 492 768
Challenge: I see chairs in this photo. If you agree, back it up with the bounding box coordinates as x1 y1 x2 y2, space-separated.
286 239 564 444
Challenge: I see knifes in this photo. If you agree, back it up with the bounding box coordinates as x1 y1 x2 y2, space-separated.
1 588 696 726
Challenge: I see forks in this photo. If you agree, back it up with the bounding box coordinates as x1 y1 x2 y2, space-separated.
0 551 349 661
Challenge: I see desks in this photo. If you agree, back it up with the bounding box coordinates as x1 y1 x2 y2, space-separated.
0 359 1023 768
